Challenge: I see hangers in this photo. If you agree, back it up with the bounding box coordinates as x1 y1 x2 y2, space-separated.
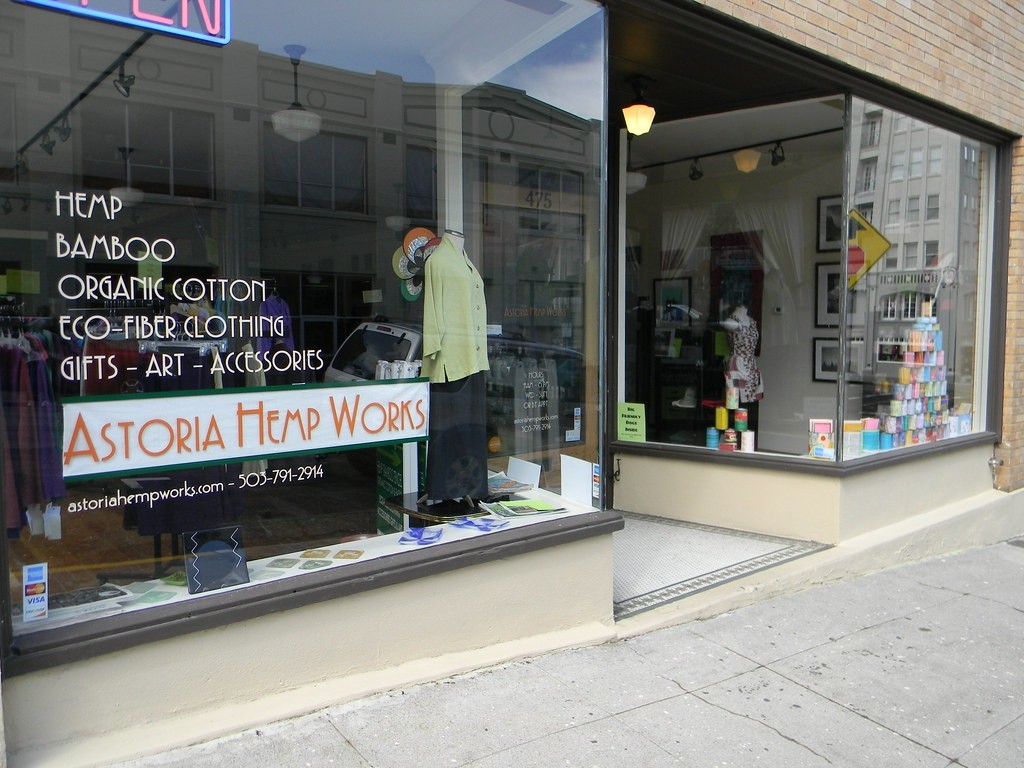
0 302 32 354
91 282 284 356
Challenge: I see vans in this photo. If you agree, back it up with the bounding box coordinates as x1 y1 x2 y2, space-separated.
325 319 597 428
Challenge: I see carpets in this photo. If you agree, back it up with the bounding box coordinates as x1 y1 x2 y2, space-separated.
613 509 836 622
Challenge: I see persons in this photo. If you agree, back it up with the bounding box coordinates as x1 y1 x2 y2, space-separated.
723 306 764 403
864 363 872 372
419 229 490 500
946 368 972 382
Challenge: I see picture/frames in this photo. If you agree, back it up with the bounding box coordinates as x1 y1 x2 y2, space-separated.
815 261 866 328
812 337 864 384
653 277 692 328
817 194 860 253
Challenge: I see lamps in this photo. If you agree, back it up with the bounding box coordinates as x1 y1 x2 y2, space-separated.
53 116 72 142
385 184 410 232
16 151 28 175
113 62 135 98
270 44 323 141
689 157 704 180
110 147 145 208
733 148 761 172
626 132 647 195
39 129 55 156
622 75 656 136
769 140 785 165
1 198 12 215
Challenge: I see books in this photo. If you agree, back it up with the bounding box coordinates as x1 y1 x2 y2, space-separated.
478 500 567 519
